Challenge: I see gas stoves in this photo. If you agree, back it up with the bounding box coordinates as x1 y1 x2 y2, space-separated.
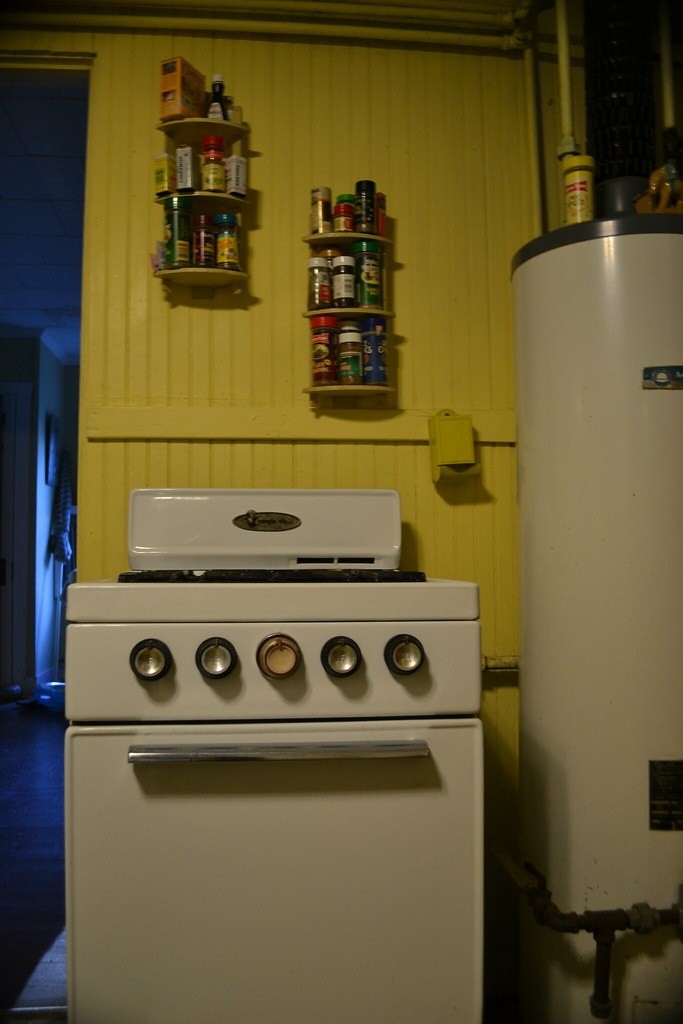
63 487 482 717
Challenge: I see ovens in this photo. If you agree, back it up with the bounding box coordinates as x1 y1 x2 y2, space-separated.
63 724 485 1023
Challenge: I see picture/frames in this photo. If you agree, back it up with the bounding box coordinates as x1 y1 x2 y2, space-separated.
44 412 70 489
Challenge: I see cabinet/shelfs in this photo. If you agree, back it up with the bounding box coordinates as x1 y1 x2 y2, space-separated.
153 117 398 400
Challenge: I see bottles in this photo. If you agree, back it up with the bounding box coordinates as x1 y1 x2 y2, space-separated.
198 135 226 192
206 74 227 121
163 197 191 269
307 257 332 310
154 152 175 196
361 317 390 387
355 180 377 235
562 154 597 228
310 317 339 387
175 141 198 192
311 186 333 235
191 214 217 268
318 250 343 268
224 154 248 197
353 242 383 311
333 204 354 233
330 256 357 309
337 321 364 385
214 213 242 271
